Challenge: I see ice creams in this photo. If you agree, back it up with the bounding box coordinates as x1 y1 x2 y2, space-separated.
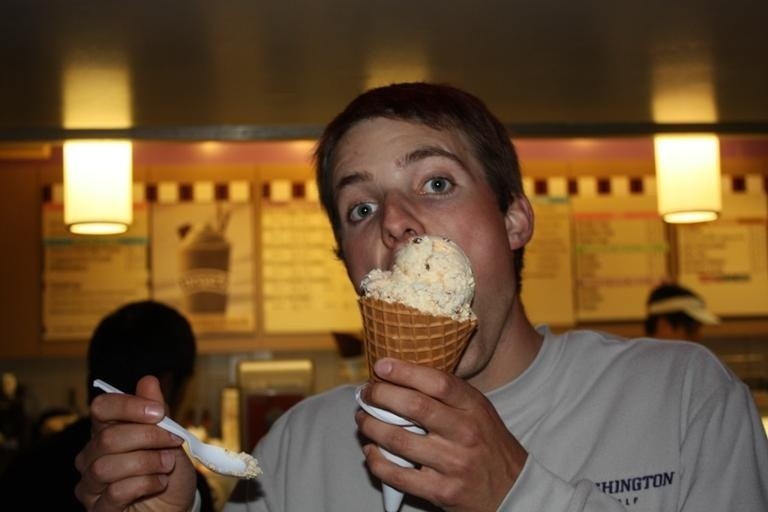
358 234 480 410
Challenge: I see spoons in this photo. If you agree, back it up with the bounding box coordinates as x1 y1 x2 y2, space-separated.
93 379 259 478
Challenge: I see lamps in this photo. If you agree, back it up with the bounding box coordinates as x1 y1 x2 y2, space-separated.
652 134 724 224
62 137 136 237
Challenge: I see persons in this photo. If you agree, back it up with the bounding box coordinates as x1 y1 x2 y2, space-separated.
7 297 218 511
73 79 767 510
644 279 720 344
